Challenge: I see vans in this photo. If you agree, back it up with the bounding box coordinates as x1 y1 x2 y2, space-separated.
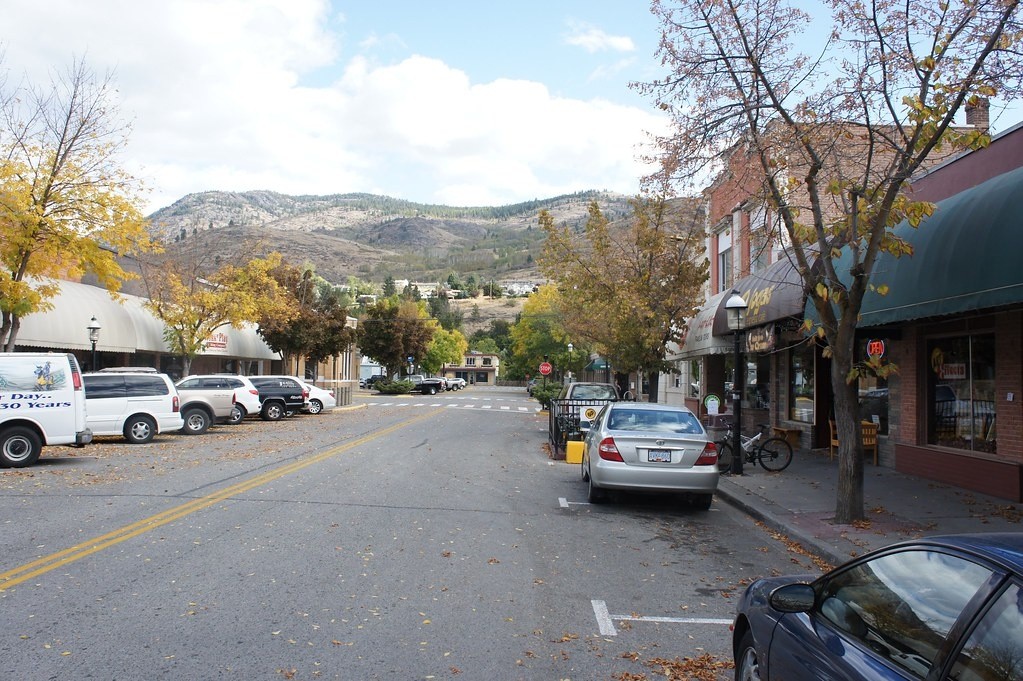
0 347 93 469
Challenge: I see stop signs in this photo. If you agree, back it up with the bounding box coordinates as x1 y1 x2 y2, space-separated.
539 362 552 375
525 374 530 379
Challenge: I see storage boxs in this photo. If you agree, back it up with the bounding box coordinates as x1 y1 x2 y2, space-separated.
566 441 584 464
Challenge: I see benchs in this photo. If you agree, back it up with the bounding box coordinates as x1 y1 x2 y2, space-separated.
772 426 802 446
828 418 879 466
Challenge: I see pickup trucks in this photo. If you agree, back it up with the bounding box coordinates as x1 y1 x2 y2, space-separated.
95 364 238 437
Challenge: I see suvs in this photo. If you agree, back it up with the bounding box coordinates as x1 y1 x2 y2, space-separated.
244 373 310 418
81 371 185 444
173 373 263 426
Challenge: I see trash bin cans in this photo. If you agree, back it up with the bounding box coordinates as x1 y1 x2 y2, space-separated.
707 413 734 465
701 414 709 429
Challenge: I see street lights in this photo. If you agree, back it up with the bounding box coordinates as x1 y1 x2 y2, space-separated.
86 315 102 373
567 342 573 382
721 285 750 476
386 362 389 378
380 366 384 392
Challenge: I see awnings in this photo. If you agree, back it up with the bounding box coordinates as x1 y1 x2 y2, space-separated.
656 166 1023 362
0 266 284 360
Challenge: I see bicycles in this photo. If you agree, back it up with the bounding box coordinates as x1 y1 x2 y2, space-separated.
712 418 794 475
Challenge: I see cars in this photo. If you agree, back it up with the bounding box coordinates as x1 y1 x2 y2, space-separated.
359 372 468 396
729 530 1023 681
861 626 900 658
554 381 622 432
888 651 953 681
860 382 959 428
526 377 550 397
245 377 305 421
579 400 720 510
295 383 337 415
